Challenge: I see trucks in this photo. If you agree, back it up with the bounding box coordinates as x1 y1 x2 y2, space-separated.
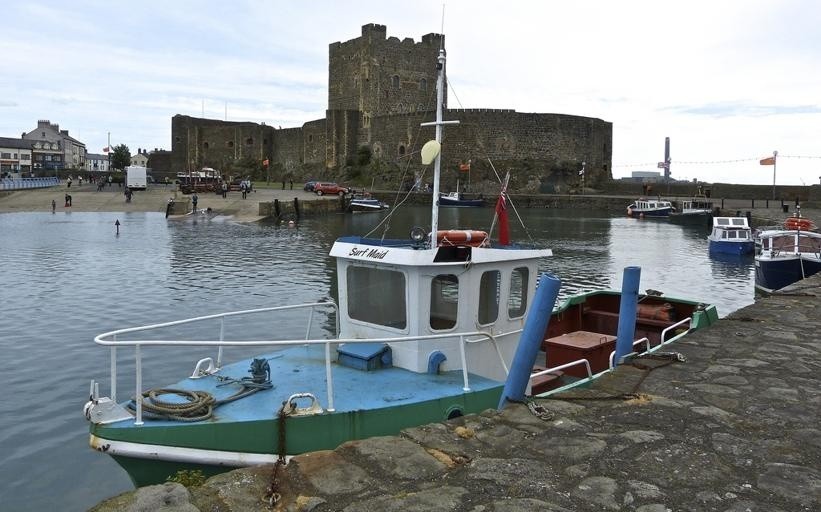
123 163 147 189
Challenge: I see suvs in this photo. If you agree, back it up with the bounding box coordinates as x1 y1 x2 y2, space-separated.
311 180 348 197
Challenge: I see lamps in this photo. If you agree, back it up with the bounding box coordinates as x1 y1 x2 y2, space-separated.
410 227 426 245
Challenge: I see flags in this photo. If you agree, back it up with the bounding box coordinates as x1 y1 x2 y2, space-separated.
103 147 109 152
760 157 776 165
460 164 470 171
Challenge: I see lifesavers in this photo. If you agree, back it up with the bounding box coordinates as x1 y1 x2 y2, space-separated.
428 229 488 242
783 218 811 230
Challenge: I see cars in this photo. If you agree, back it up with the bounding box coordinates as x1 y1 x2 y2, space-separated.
303 181 316 193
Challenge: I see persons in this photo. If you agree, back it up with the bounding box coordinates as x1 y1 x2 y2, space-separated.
124 186 133 202
67 174 112 192
167 198 173 207
240 180 256 200
52 199 56 210
222 181 227 199
192 193 198 213
164 175 169 186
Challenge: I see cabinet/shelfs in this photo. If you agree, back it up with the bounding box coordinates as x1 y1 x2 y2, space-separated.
545 331 616 379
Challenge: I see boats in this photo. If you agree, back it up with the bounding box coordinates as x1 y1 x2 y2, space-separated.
665 181 719 228
174 164 224 184
623 181 676 219
438 174 488 209
746 199 820 297
71 2 726 496
706 210 757 260
344 186 388 214
